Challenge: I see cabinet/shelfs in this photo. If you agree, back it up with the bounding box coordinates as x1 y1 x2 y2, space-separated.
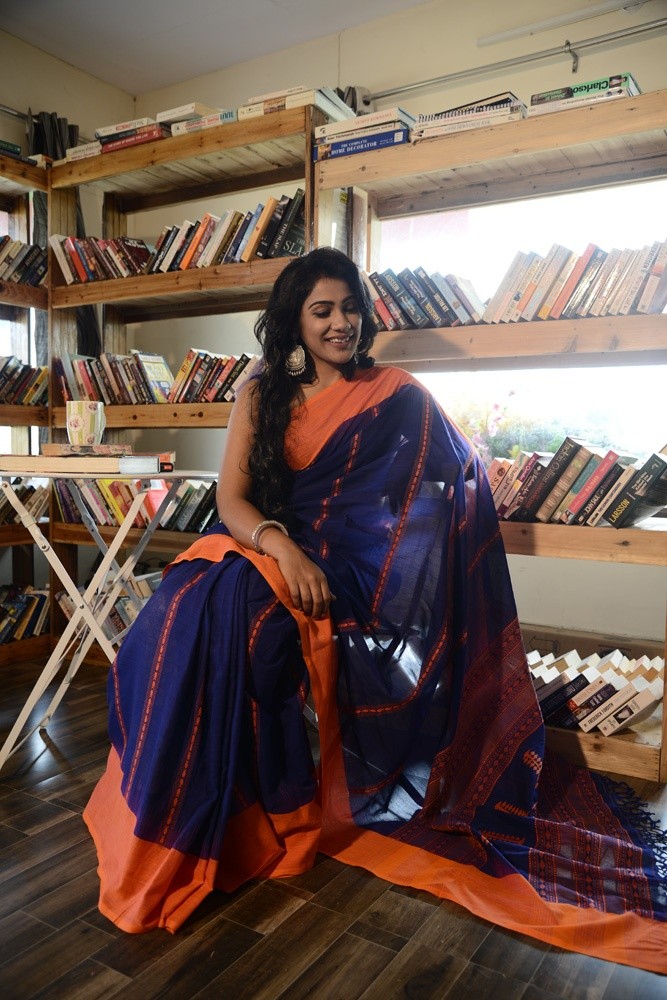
0 80 667 788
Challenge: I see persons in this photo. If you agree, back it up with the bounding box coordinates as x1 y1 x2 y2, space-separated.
82 246 543 935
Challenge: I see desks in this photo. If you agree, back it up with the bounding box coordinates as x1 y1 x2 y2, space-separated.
0 470 220 774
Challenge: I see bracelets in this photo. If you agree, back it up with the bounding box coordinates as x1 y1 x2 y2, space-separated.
252 520 289 556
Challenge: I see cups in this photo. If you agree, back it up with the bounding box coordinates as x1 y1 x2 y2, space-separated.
66 400 107 446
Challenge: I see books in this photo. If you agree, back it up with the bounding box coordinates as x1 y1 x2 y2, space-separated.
51 478 223 535
0 481 50 526
530 71 642 105
0 582 51 645
100 122 171 145
0 234 49 288
317 183 369 272
485 436 667 528
237 84 357 122
48 233 155 286
55 348 174 406
482 237 667 324
156 101 225 123
166 349 268 404
0 355 48 406
354 265 486 331
524 648 665 737
171 109 238 137
0 140 56 170
94 117 155 138
527 86 630 117
408 90 528 142
102 128 172 154
143 187 314 276
52 140 103 167
313 107 416 163
54 569 163 648
